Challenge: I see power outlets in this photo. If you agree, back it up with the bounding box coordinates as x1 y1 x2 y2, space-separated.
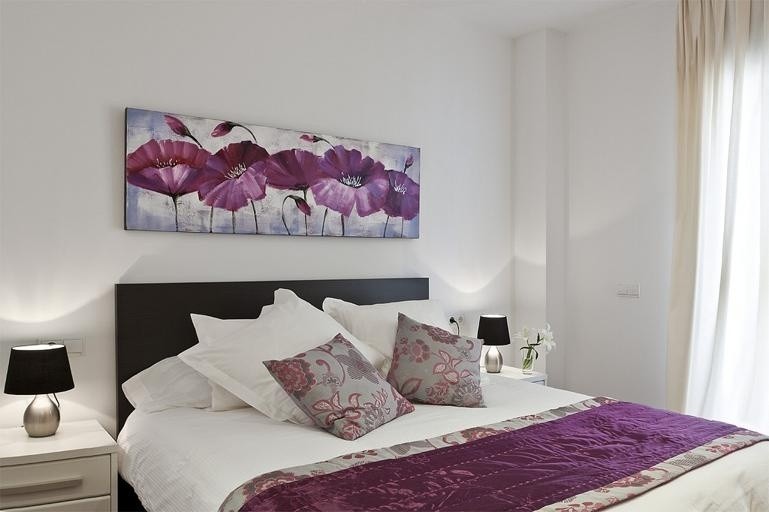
614 284 639 300
38 335 87 358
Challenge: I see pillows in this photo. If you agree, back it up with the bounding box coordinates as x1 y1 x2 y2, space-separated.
262 333 414 441
180 288 385 425
386 312 485 407
322 297 454 380
191 314 257 412
122 355 211 413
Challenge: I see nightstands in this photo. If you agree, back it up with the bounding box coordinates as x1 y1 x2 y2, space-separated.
481 364 549 387
0 418 120 512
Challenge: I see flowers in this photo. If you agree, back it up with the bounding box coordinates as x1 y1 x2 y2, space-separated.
512 322 556 359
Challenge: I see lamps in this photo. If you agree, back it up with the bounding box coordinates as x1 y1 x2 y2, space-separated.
3 345 74 436
477 313 510 372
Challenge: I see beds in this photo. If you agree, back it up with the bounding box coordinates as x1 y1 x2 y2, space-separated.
114 277 769 511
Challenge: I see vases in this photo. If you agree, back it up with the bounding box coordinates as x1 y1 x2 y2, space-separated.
521 347 534 374
